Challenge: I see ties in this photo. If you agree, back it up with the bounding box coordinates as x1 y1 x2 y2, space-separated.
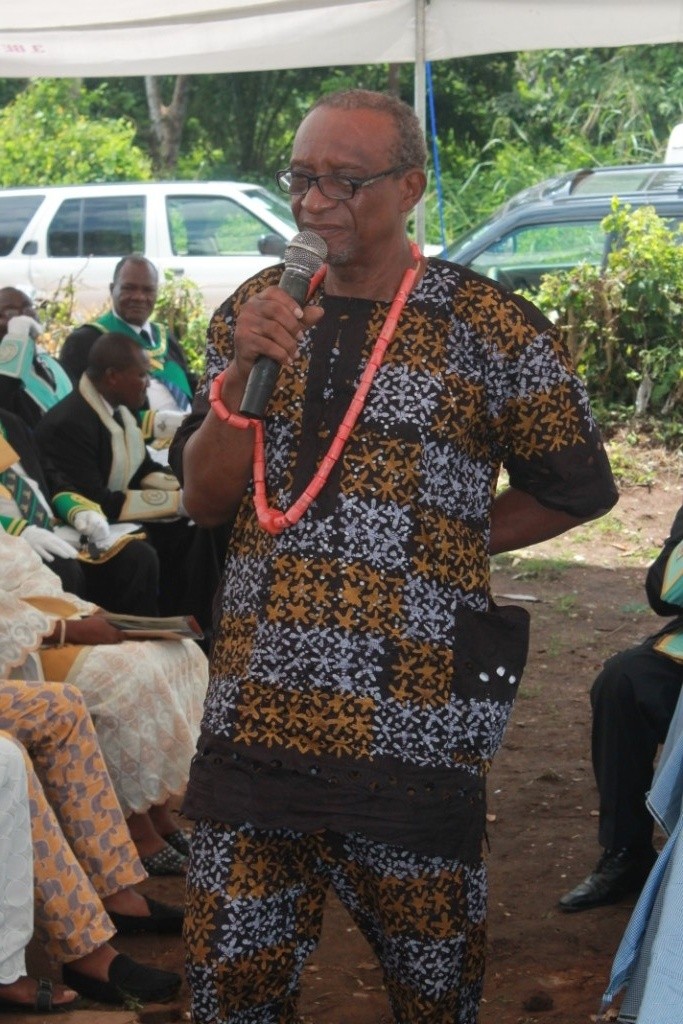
140 330 153 346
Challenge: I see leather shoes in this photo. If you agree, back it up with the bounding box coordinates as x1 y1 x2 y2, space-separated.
558 843 659 911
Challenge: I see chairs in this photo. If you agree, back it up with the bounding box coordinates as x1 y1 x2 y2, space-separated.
183 207 222 253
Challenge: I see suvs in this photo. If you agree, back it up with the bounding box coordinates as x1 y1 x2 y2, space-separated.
433 164 683 294
0 182 300 328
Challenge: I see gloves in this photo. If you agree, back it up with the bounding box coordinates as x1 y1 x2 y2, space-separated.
21 525 79 562
7 315 43 340
73 510 109 542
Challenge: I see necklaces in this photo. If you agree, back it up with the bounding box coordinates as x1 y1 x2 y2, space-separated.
252 239 423 533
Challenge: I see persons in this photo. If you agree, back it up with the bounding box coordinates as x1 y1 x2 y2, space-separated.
558 508 683 912
0 254 228 1014
167 86 621 784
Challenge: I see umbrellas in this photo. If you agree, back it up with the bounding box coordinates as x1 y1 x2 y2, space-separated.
0 1 683 250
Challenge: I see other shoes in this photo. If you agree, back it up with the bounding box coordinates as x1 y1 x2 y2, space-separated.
166 829 192 855
107 894 184 935
142 845 188 876
63 953 182 1005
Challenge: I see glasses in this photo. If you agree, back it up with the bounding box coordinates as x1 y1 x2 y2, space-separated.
277 165 408 200
0 304 38 318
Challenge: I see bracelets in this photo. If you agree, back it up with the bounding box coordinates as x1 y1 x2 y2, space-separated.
207 369 259 429
58 618 67 647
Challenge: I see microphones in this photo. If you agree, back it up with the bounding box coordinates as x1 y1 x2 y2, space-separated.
240 231 328 418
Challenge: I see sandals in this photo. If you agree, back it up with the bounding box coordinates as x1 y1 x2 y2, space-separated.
0 977 81 1013
